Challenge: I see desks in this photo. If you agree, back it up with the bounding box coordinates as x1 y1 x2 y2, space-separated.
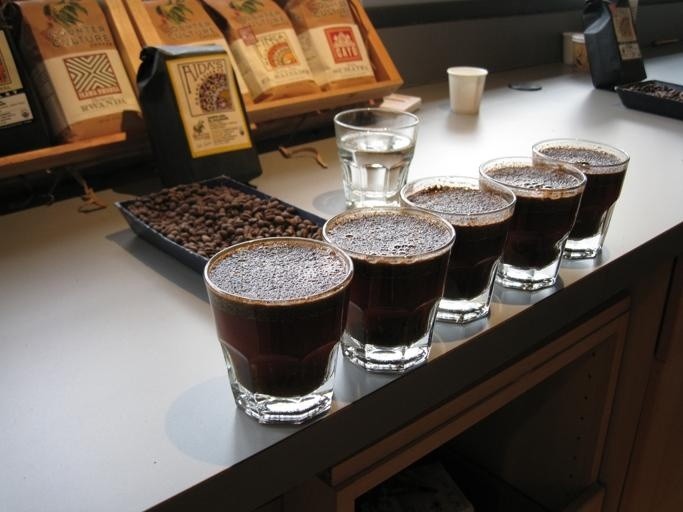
0 38 683 512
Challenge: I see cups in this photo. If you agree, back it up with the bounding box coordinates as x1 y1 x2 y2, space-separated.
398 174 517 326
203 237 355 425
447 67 488 116
531 137 630 261
563 31 590 72
334 108 419 209
321 205 456 373
477 155 586 292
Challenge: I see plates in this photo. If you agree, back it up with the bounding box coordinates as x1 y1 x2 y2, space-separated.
115 174 328 274
615 79 683 120
